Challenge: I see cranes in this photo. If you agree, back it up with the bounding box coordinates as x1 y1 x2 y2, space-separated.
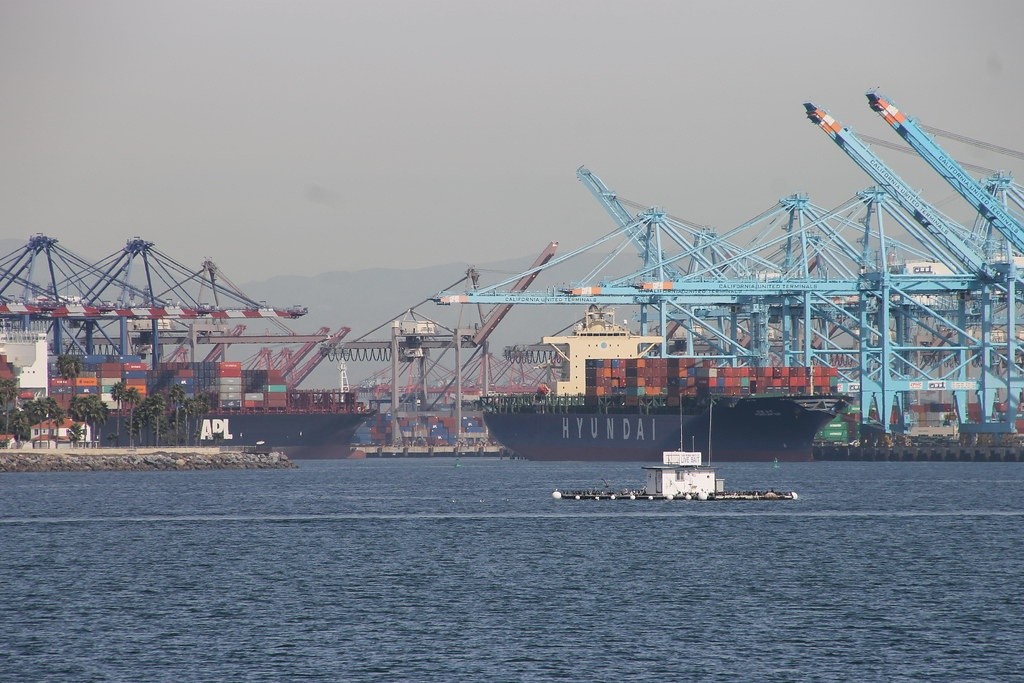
427 89 1024 454
0 231 350 363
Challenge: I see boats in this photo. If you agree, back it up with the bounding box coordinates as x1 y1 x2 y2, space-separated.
68 407 378 460
473 357 854 462
